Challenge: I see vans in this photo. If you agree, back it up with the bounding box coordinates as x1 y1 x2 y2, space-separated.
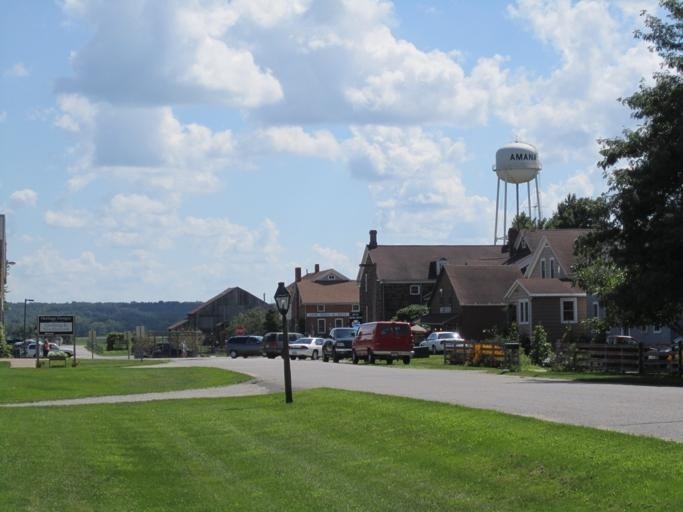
225 335 267 358
264 332 304 360
351 322 414 364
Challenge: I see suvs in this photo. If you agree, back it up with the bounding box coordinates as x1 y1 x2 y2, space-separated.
322 327 369 363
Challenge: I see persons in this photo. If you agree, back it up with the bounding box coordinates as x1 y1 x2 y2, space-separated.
42 338 49 358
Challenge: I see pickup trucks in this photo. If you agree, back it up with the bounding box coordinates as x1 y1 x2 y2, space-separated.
556 335 640 372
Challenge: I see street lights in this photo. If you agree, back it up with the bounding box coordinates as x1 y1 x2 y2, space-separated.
274 282 292 403
24 299 34 355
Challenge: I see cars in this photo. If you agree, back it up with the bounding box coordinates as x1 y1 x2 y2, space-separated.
7 337 74 358
288 337 324 360
420 332 468 354
143 342 195 357
